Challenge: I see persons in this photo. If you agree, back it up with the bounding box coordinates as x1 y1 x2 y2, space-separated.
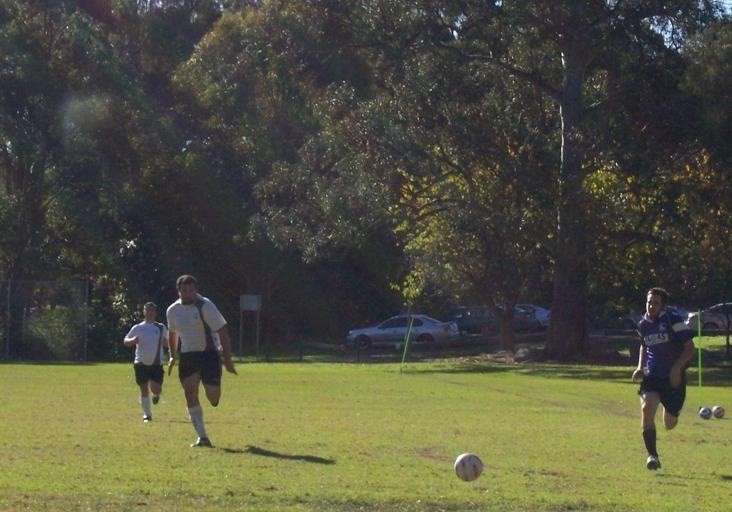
124 302 168 424
167 275 238 448
631 287 696 471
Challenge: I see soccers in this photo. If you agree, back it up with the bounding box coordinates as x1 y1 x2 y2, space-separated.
699 408 711 419
713 407 723 417
454 453 483 481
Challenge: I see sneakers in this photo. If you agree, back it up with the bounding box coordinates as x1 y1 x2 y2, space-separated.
143 413 153 423
645 454 661 471
152 393 160 405
210 400 219 407
190 437 213 448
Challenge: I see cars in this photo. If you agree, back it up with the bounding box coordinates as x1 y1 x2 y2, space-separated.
346 303 551 351
614 303 732 335
